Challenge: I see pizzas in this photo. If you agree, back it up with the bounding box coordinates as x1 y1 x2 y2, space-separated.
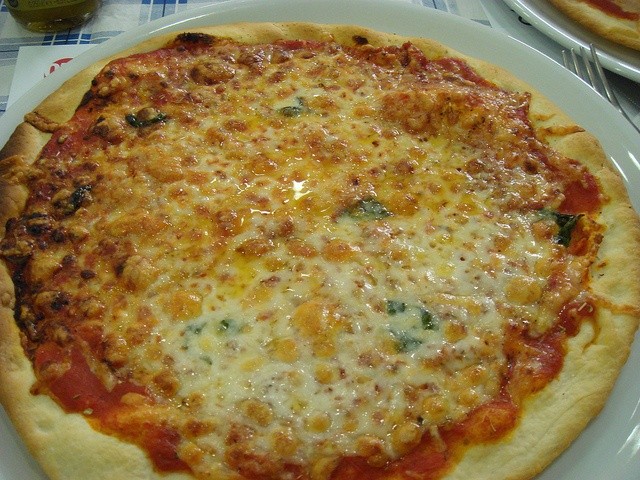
548 0 640 51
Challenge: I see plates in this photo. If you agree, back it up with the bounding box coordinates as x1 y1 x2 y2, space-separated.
503 2 640 82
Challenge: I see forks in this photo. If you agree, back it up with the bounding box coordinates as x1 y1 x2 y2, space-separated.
556 44 640 141
